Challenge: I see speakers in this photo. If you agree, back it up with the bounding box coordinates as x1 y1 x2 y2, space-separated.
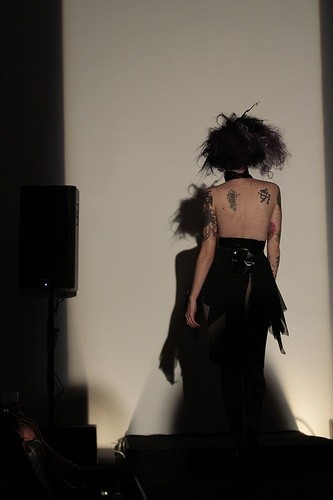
18 185 76 289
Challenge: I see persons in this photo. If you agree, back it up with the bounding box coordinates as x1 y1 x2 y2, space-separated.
184 103 290 437
